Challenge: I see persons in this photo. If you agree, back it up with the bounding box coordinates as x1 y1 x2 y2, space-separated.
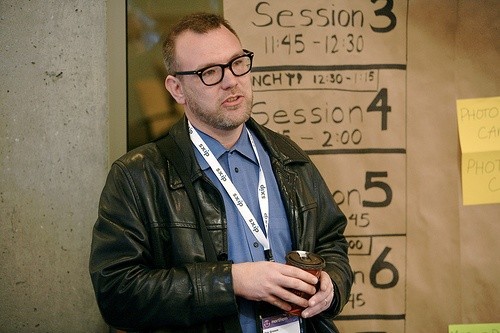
87 11 355 333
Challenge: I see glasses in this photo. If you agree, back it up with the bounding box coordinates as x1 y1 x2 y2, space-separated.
167 47 254 86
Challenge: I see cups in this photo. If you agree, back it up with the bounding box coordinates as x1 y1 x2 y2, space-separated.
284 251 326 316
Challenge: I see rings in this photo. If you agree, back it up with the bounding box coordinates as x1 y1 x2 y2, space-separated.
324 298 328 305
